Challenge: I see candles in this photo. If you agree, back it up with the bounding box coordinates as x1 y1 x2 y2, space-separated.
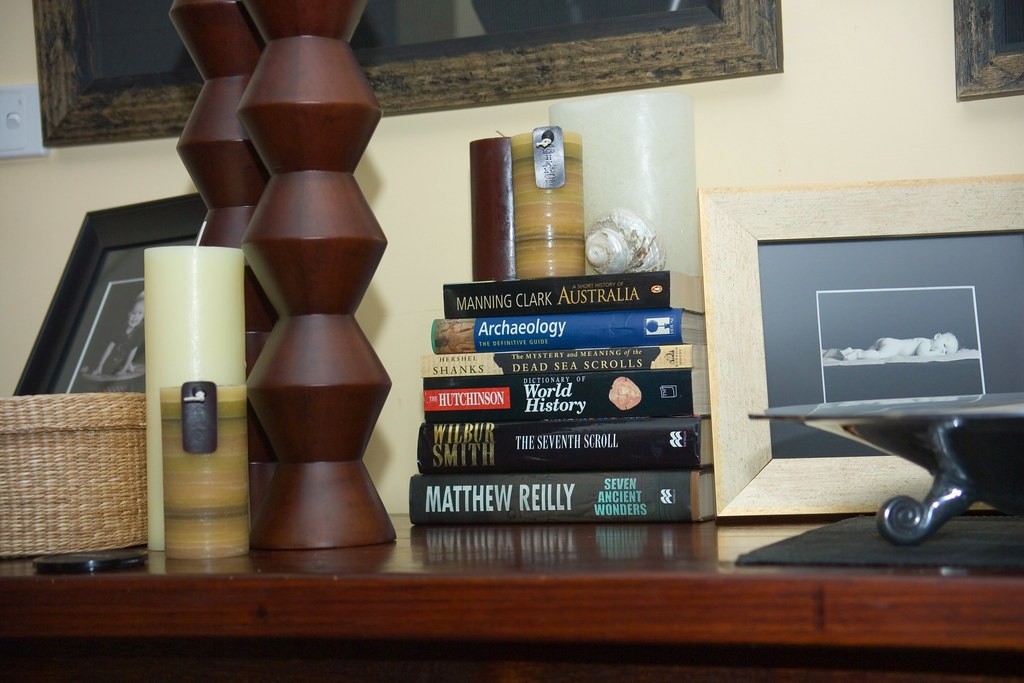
145 240 251 562
163 380 251 562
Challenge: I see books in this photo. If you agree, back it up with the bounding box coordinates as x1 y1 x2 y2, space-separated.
407 270 716 526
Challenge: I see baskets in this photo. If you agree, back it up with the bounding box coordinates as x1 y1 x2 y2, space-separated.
0 393 148 558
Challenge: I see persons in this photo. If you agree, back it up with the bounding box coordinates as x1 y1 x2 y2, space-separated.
839 332 959 361
89 291 145 378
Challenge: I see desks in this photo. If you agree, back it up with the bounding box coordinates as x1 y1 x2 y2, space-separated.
0 514 1024 683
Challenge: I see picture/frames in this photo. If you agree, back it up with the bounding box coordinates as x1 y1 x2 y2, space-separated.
699 171 1024 518
32 0 785 151
11 189 212 399
